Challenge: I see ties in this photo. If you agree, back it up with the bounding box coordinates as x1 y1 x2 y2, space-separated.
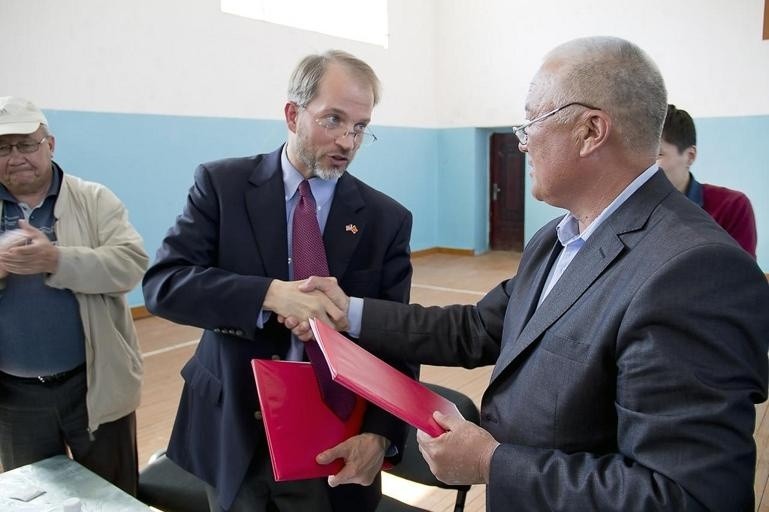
293 181 356 422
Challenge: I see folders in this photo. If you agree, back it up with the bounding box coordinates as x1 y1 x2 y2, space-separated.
251 359 393 482
309 317 466 438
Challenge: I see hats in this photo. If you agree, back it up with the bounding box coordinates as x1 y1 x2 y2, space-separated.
0 96 48 136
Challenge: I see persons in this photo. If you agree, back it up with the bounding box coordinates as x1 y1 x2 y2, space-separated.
142 48 415 512
0 94 151 498
278 34 768 512
658 104 758 266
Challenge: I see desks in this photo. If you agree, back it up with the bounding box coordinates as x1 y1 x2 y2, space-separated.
0 456 161 512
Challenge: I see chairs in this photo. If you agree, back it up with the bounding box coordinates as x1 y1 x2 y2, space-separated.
136 450 209 511
377 382 481 512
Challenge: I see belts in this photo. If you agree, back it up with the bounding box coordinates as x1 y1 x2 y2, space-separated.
0 361 86 385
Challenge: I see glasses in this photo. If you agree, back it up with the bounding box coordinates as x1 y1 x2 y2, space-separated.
304 106 378 146
0 136 47 156
512 102 601 145
